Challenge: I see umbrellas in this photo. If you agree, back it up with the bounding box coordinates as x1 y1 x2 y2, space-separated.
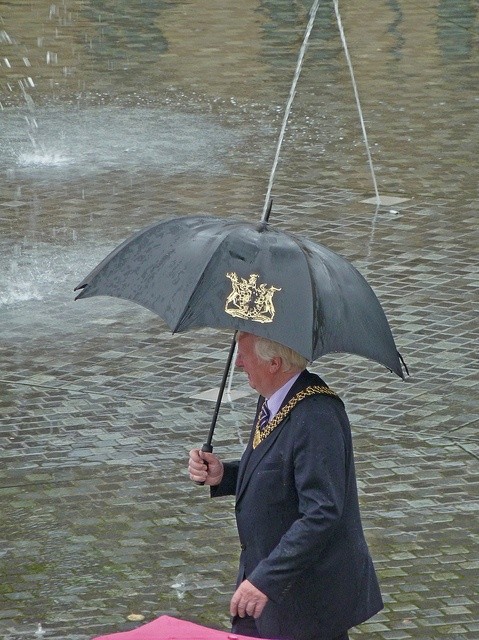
73 198 410 486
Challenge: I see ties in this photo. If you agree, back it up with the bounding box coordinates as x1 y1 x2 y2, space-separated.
259 403 270 434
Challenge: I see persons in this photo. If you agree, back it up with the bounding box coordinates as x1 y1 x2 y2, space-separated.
187 330 384 640
